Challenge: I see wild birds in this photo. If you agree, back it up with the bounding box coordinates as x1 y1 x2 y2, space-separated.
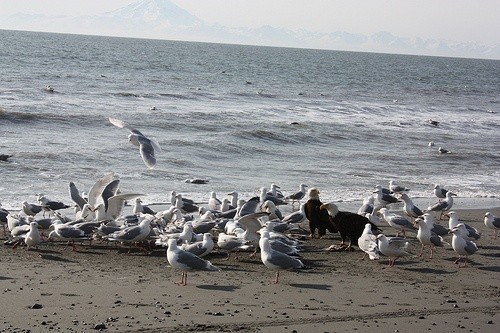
47 85 54 91
108 117 162 169
256 89 263 94
428 140 451 154
0 171 500 286
